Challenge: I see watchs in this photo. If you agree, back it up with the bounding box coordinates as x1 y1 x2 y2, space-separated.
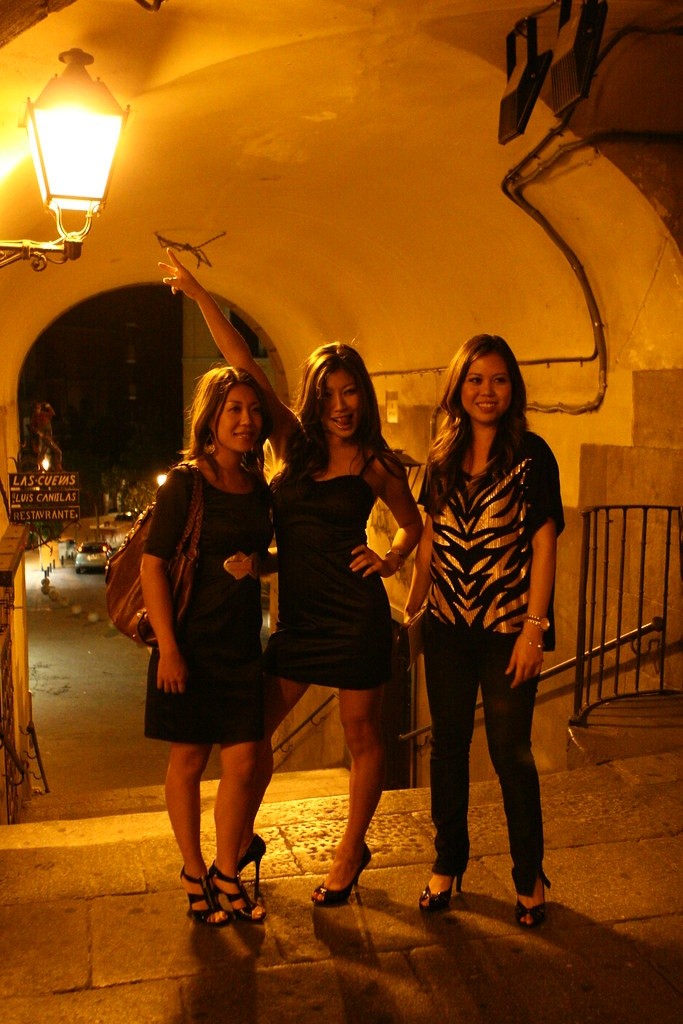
523 614 550 632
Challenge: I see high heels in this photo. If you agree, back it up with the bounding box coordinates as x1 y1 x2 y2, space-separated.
311 842 371 906
514 877 546 928
179 865 233 927
208 859 267 923
237 833 266 889
418 864 467 912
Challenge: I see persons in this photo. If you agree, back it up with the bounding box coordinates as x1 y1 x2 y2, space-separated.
404 334 566 930
31 399 62 472
158 247 424 905
141 366 274 927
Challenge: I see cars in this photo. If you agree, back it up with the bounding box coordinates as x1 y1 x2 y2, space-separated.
74 540 115 575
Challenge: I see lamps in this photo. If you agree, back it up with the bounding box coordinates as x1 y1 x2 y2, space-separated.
497 0 610 150
0 45 134 272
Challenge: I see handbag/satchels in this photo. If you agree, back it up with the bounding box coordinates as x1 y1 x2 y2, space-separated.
103 460 204 649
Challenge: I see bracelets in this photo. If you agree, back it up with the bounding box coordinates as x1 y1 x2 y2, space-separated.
385 547 405 570
521 632 542 650
